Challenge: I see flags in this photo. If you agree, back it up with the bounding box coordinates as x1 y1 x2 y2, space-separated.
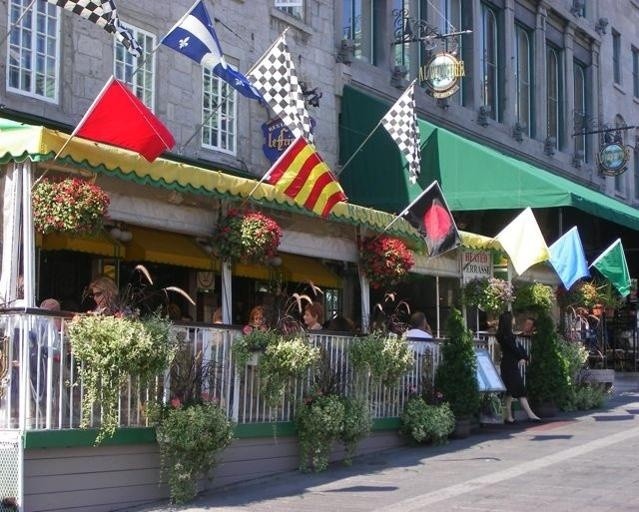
74 76 175 162
163 2 254 99
266 136 348 217
404 181 464 260
548 227 590 291
496 205 549 276
592 239 634 299
248 34 312 143
45 1 142 56
382 83 423 184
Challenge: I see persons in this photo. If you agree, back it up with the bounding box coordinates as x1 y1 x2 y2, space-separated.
402 313 434 356
0 277 132 397
165 303 224 344
521 318 537 336
303 301 324 329
249 305 271 328
495 312 546 423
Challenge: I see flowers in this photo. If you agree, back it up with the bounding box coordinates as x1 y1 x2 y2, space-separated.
32 175 111 236
211 209 284 266
355 234 414 289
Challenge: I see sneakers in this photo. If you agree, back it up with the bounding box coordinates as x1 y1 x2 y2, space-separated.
528 417 549 425
504 419 523 426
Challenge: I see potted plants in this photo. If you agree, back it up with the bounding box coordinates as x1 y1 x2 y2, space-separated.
70 308 579 508
584 285 620 318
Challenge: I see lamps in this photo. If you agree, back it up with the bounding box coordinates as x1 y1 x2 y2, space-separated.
272 256 282 266
112 220 133 243
